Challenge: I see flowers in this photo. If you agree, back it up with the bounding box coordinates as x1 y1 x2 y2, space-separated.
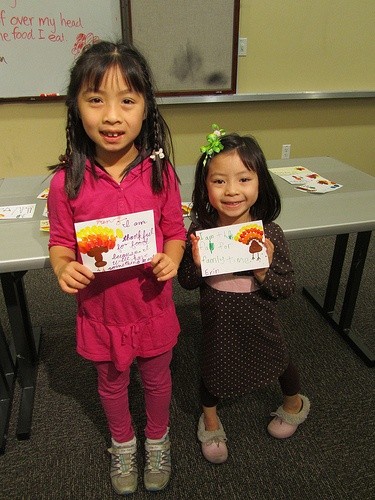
200 124 225 167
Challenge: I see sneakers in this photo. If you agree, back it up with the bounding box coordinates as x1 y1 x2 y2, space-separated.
107 434 138 495
143 426 172 491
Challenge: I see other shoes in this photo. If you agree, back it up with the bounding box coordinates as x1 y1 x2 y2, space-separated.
198 412 228 464
267 394 310 439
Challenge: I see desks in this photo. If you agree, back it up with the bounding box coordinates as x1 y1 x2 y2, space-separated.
0 157 375 454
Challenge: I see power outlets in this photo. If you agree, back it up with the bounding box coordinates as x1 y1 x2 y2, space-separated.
282 144 291 159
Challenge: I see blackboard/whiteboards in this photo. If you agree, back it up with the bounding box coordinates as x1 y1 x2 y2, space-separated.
0 0 130 102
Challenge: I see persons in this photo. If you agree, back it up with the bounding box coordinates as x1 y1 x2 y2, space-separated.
178 133 311 461
47 42 186 494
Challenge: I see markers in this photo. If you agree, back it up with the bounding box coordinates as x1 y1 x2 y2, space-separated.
40 93 59 97
